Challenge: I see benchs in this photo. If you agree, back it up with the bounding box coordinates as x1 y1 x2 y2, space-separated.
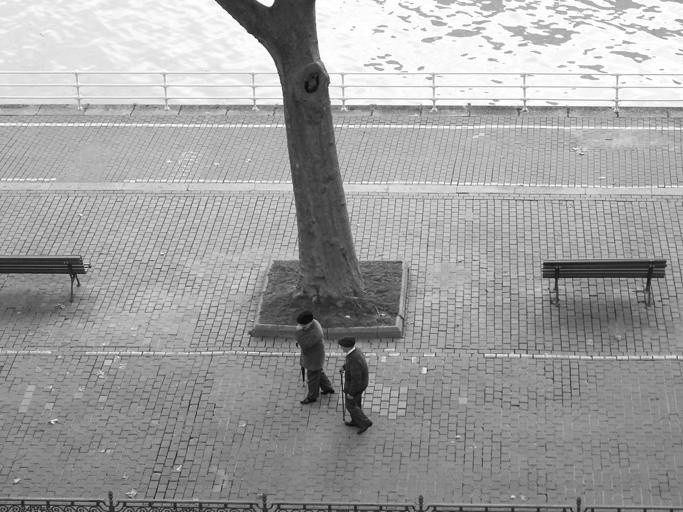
539 255 666 310
0 255 90 305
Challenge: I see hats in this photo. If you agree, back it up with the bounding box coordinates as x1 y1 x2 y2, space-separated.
338 336 355 347
297 311 313 324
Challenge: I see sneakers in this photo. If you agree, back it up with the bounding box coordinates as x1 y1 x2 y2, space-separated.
345 420 372 434
300 389 334 404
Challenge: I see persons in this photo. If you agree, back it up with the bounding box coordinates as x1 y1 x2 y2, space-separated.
338 336 372 435
294 311 335 403
